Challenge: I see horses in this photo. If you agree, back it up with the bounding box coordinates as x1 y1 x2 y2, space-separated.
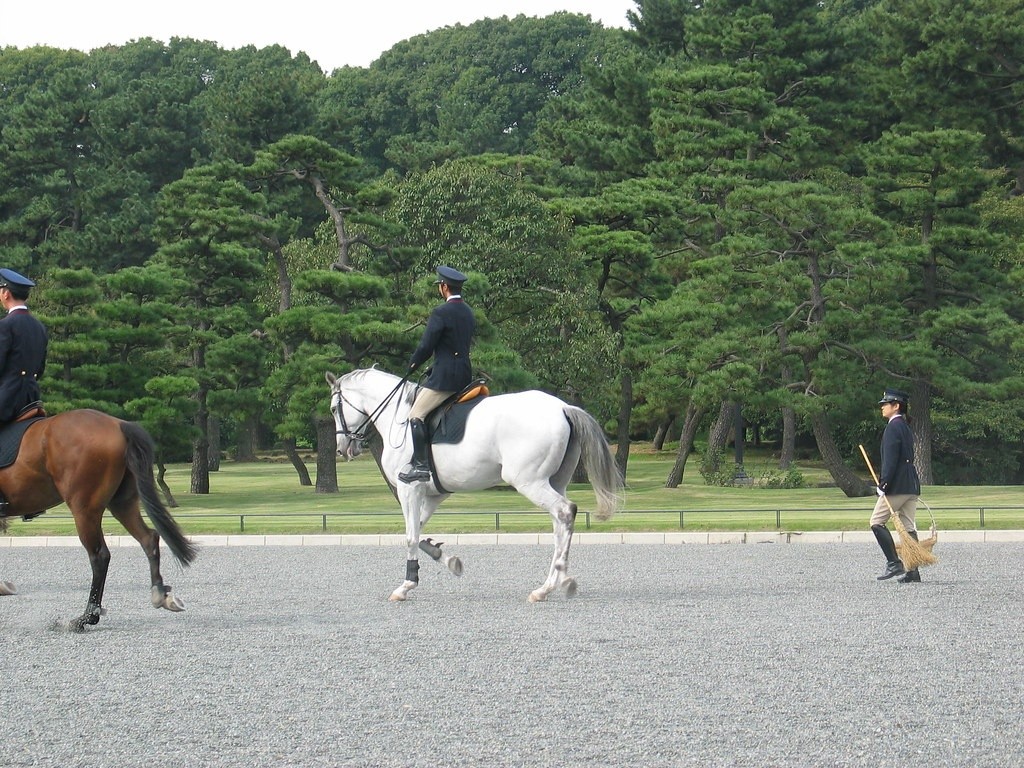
0 408 201 633
324 363 627 603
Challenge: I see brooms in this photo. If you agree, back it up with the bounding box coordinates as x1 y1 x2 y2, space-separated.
858 444 938 572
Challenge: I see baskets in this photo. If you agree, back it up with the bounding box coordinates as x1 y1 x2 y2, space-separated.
895 498 937 561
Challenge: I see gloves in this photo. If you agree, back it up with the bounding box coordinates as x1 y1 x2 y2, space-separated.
876 487 885 497
425 366 432 376
406 363 418 375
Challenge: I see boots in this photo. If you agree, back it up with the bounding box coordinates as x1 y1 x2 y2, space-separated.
897 530 921 583
871 525 905 580
398 417 430 484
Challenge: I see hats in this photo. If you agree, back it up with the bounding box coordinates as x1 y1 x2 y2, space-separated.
0 268 35 293
433 265 468 287
877 388 910 405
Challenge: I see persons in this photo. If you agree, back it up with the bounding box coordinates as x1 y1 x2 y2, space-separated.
0 268 46 522
394 266 474 484
868 389 921 582
